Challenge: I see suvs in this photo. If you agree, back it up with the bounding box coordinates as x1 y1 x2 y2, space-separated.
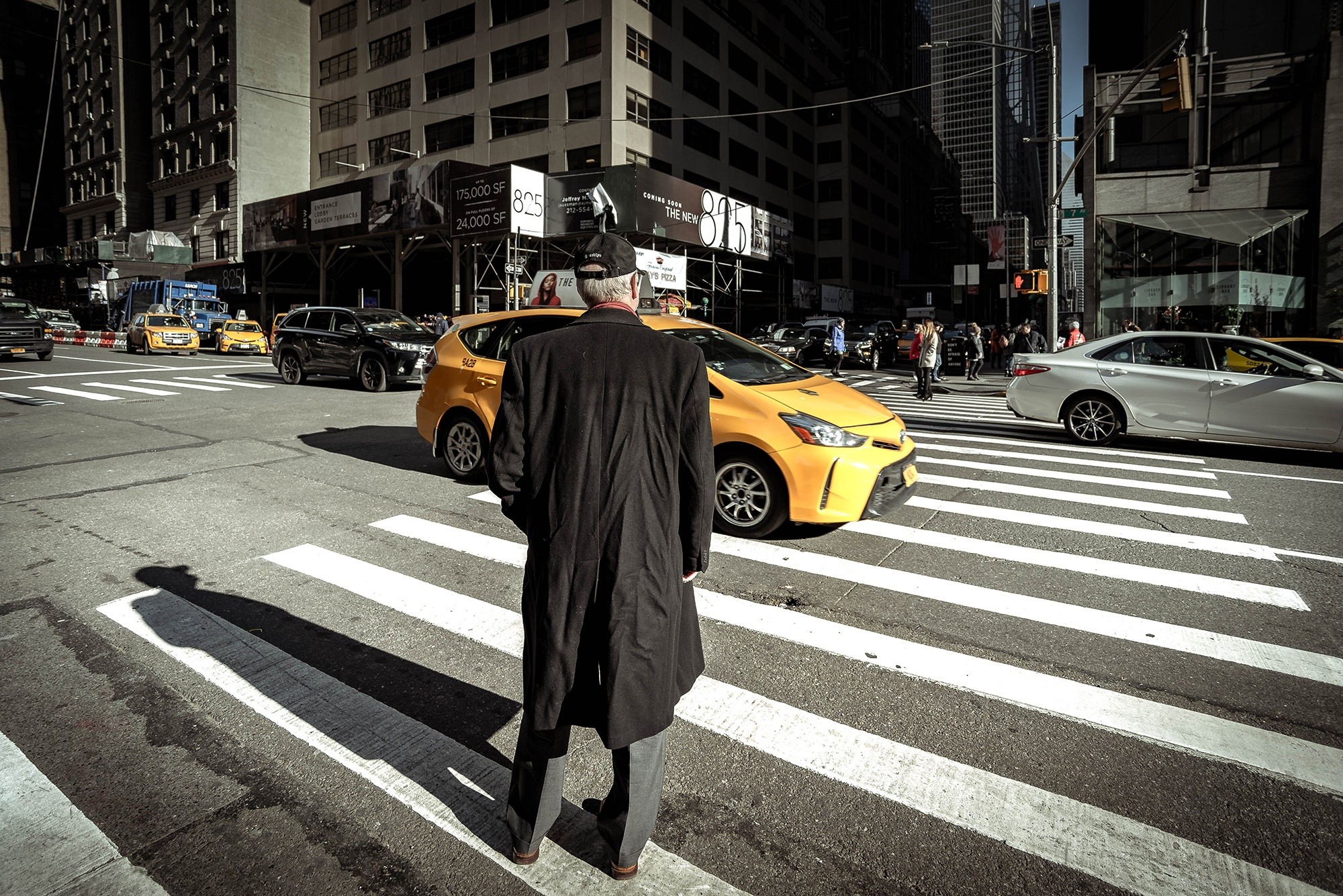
0 297 55 362
271 307 443 393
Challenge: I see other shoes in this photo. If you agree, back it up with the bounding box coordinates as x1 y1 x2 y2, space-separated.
600 794 639 880
834 371 845 378
913 392 921 397
932 378 939 383
967 376 977 381
1008 372 1013 377
939 377 949 381
974 376 980 380
513 844 539 865
1005 371 1008 377
831 369 835 376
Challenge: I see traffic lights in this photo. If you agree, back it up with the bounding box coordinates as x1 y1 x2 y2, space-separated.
1012 272 1036 292
518 285 532 300
1158 58 1194 113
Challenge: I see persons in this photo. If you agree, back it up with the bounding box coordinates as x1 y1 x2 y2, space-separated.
531 273 561 306
930 321 946 383
1216 348 1268 376
1225 327 1261 339
965 322 1048 381
1063 321 1087 348
909 323 925 397
1122 319 1142 333
830 316 845 378
484 231 717 879
917 318 939 402
434 313 449 337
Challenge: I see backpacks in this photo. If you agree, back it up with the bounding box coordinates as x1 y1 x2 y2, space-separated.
999 331 1010 348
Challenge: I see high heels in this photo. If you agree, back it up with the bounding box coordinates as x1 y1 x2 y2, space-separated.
918 393 934 401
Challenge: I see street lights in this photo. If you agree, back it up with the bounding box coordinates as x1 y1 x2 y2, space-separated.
1005 210 1023 332
918 40 1049 232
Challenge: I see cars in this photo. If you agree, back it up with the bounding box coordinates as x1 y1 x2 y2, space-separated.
745 312 995 370
270 312 289 351
30 307 81 331
394 322 434 333
215 309 267 357
1003 328 1343 457
125 303 200 356
413 268 919 541
1225 338 1343 377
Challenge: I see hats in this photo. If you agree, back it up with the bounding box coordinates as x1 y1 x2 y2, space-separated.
972 323 981 333
573 232 646 281
837 317 844 324
1002 322 1011 329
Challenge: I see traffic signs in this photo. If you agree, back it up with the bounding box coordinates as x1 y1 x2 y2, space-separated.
509 255 528 264
1031 233 1075 249
504 262 524 275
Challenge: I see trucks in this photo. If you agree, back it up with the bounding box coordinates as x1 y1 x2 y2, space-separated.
117 276 232 342
